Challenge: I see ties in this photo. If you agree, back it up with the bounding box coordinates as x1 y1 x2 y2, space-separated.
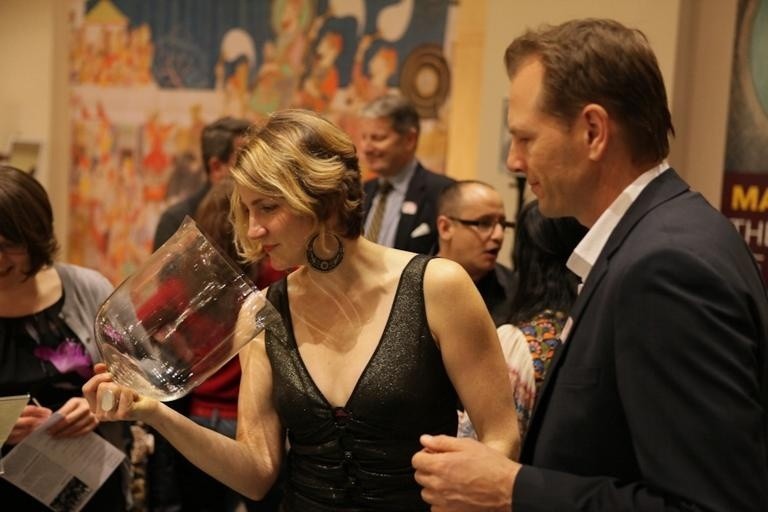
366 180 390 242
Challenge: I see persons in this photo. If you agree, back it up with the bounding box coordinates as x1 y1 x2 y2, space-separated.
152 117 260 253
135 176 300 512
80 109 523 511
1 165 158 512
427 179 519 330
457 199 592 464
410 16 768 511
357 95 459 256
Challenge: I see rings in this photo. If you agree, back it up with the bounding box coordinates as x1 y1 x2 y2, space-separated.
100 390 118 411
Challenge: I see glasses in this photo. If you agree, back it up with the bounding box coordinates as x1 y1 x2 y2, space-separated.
449 215 517 236
